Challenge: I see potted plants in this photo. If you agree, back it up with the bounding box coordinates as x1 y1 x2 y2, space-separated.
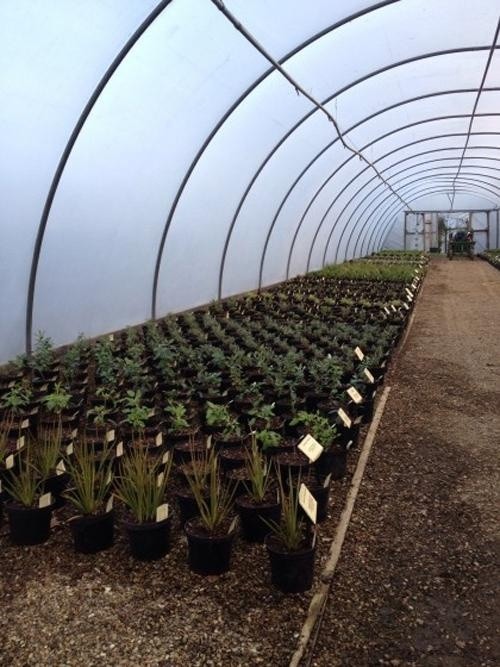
0 250 428 593
